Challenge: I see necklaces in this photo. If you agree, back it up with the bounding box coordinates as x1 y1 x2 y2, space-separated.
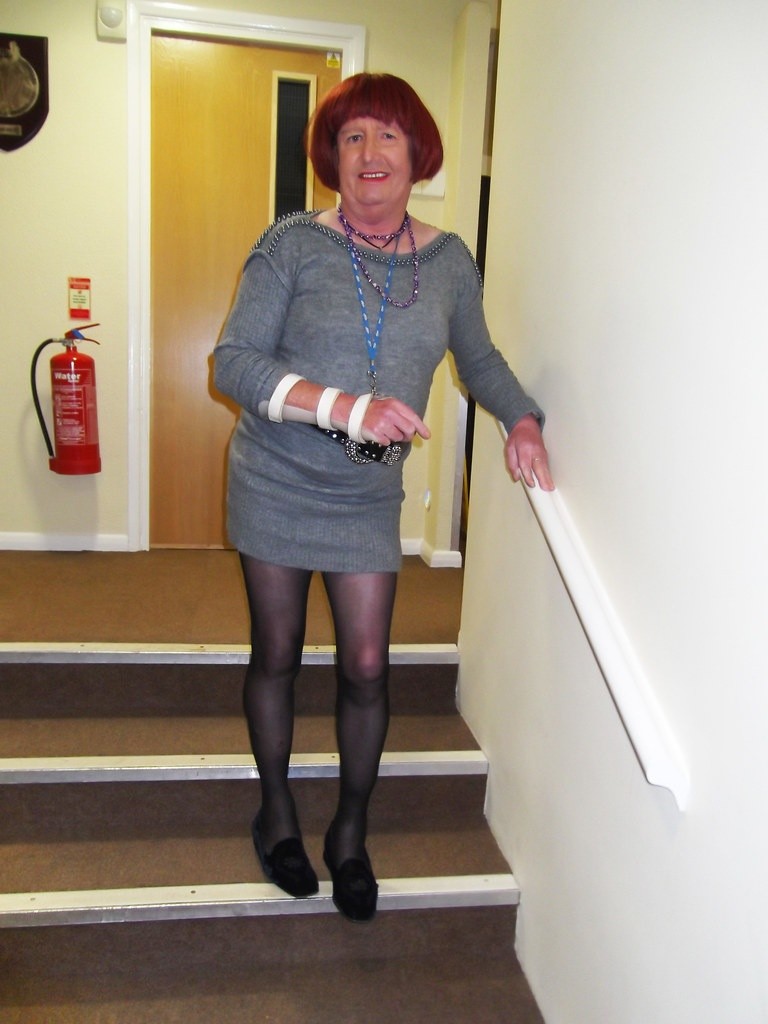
337 203 419 308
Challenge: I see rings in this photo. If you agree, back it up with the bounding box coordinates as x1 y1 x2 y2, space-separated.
533 458 539 461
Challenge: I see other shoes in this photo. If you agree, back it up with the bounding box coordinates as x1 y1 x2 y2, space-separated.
322 819 379 923
250 815 319 899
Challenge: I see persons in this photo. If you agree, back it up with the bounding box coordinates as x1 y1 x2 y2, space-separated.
212 72 555 921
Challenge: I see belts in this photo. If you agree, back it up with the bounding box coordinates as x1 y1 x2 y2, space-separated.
309 423 410 466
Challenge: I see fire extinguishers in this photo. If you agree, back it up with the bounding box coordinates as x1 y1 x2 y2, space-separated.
30 322 103 476
